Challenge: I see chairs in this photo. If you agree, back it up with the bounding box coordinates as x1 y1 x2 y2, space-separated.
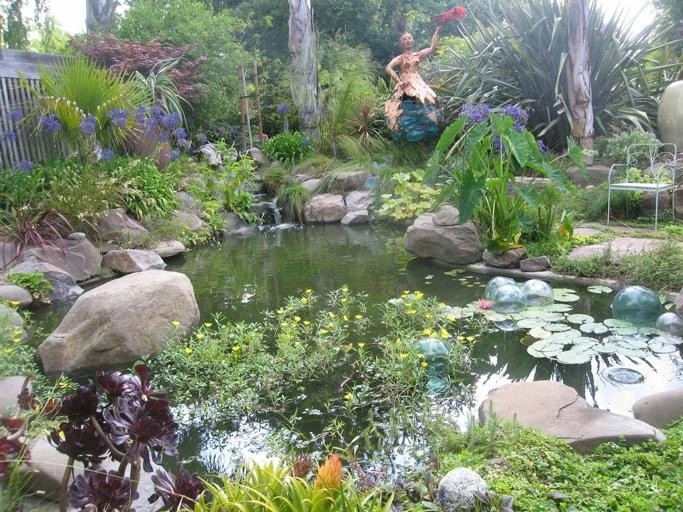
607 143 677 231
